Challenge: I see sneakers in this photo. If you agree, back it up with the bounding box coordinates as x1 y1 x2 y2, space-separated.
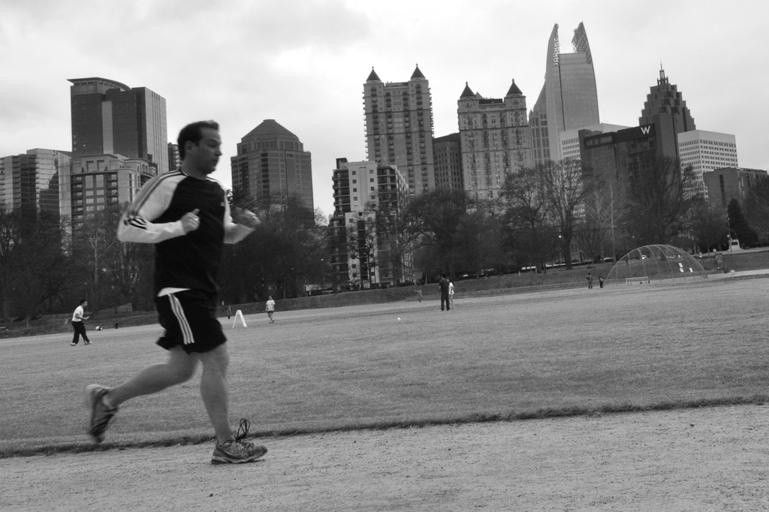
84 382 116 444
209 437 268 464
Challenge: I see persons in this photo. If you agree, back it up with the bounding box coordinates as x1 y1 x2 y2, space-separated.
438 273 455 312
264 296 276 324
715 252 725 270
586 272 594 290
412 281 424 304
70 298 92 346
598 273 604 289
82 120 268 466
